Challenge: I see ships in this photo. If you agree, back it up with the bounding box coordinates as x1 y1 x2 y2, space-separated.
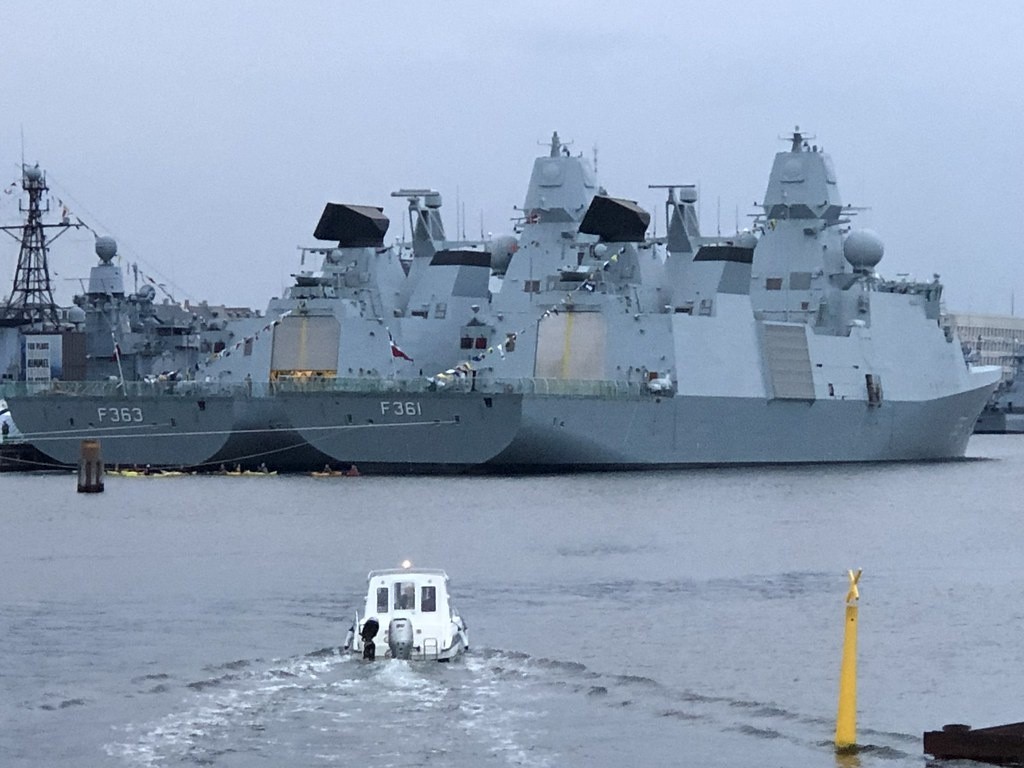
961 340 1024 434
0 123 1005 474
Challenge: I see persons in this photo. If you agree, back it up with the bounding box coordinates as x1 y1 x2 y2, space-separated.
400 587 414 607
144 372 166 385
245 374 252 396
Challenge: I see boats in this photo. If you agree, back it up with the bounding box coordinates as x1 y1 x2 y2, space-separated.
344 562 471 665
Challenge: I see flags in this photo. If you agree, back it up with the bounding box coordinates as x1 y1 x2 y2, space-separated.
390 335 414 361
427 247 626 387
164 308 293 379
